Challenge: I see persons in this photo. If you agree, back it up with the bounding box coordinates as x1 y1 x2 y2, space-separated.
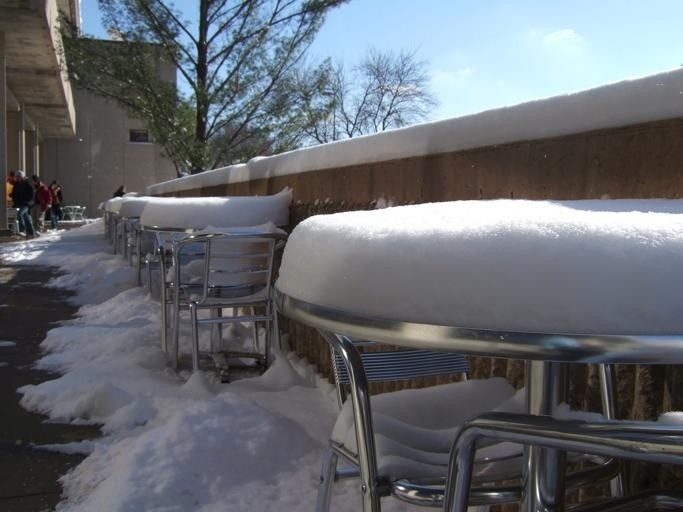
114 186 126 198
6 170 63 239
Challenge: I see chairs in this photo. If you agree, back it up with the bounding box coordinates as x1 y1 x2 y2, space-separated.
103 210 295 387
269 287 683 509
60 206 87 220
7 208 19 238
